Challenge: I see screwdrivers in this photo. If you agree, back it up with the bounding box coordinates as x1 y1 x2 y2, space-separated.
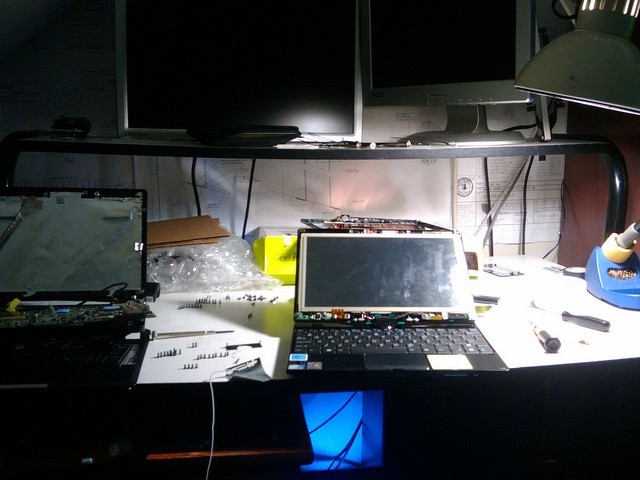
529 300 610 333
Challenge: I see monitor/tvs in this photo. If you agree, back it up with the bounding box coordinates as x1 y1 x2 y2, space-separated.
116 1 362 147
0 185 147 306
362 0 537 148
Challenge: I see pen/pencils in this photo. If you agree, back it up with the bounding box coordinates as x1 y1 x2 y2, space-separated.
528 320 561 353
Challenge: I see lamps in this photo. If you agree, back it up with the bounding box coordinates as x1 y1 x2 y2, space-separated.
514 0 640 311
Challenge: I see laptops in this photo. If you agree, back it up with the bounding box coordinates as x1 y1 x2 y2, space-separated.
287 228 512 378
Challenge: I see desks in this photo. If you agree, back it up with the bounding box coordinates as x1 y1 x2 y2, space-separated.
4 124 637 475
